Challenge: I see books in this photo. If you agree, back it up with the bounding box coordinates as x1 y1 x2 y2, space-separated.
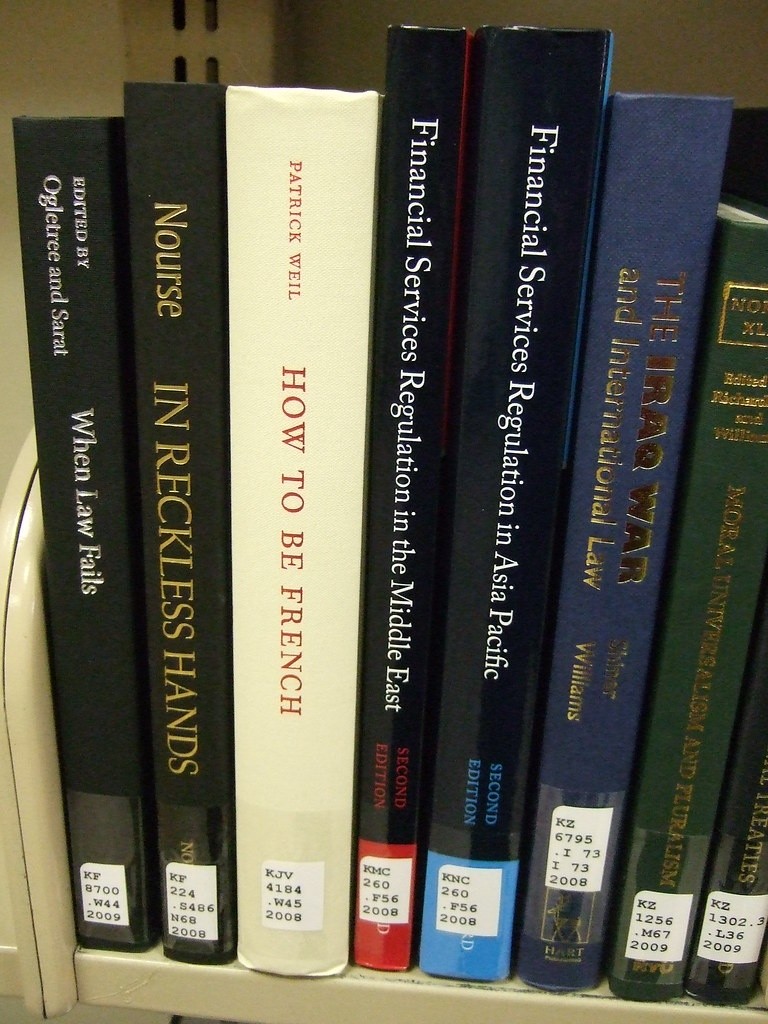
10 23 768 1007
